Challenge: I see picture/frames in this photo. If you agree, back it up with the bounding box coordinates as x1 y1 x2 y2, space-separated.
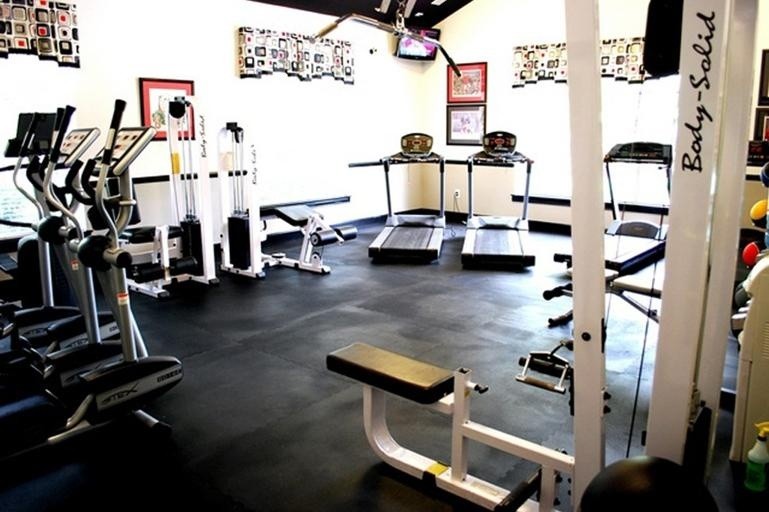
447 62 487 103
446 106 487 145
138 77 196 142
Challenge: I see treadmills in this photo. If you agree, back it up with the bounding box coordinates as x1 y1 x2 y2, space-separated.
461 130 535 268
553 141 673 278
368 132 445 263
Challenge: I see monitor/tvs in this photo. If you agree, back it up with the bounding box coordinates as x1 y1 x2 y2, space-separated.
396 28 441 61
